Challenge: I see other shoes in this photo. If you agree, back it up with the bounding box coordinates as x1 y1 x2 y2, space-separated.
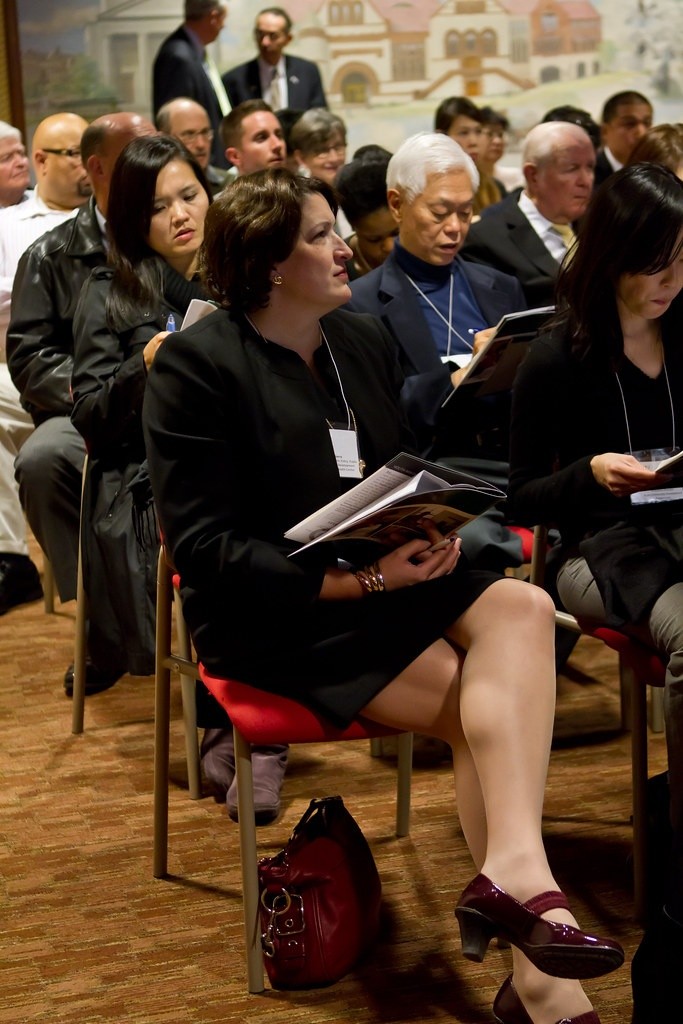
64 646 129 697
227 741 291 826
200 725 237 803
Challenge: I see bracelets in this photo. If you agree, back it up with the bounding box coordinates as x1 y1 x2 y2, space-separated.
360 561 378 593
372 556 385 592
345 566 372 595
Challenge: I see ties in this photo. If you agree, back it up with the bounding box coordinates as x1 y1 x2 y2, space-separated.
270 70 281 110
552 224 579 255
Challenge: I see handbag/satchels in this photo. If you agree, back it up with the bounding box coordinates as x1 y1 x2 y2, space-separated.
255 794 390 992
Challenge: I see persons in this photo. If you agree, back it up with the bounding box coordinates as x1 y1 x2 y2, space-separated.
502 163 683 858
220 7 329 143
140 168 626 1024
151 0 234 168
1 91 683 824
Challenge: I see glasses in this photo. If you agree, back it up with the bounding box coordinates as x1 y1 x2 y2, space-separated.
314 140 348 159
42 147 83 158
177 128 215 143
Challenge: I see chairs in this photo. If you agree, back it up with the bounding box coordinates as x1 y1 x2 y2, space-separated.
66 379 668 994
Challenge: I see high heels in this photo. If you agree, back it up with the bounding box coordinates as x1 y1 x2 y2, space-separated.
488 973 602 1024
454 871 625 980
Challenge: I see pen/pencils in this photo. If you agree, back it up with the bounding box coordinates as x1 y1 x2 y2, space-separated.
165 314 176 331
468 328 484 334
428 537 455 551
